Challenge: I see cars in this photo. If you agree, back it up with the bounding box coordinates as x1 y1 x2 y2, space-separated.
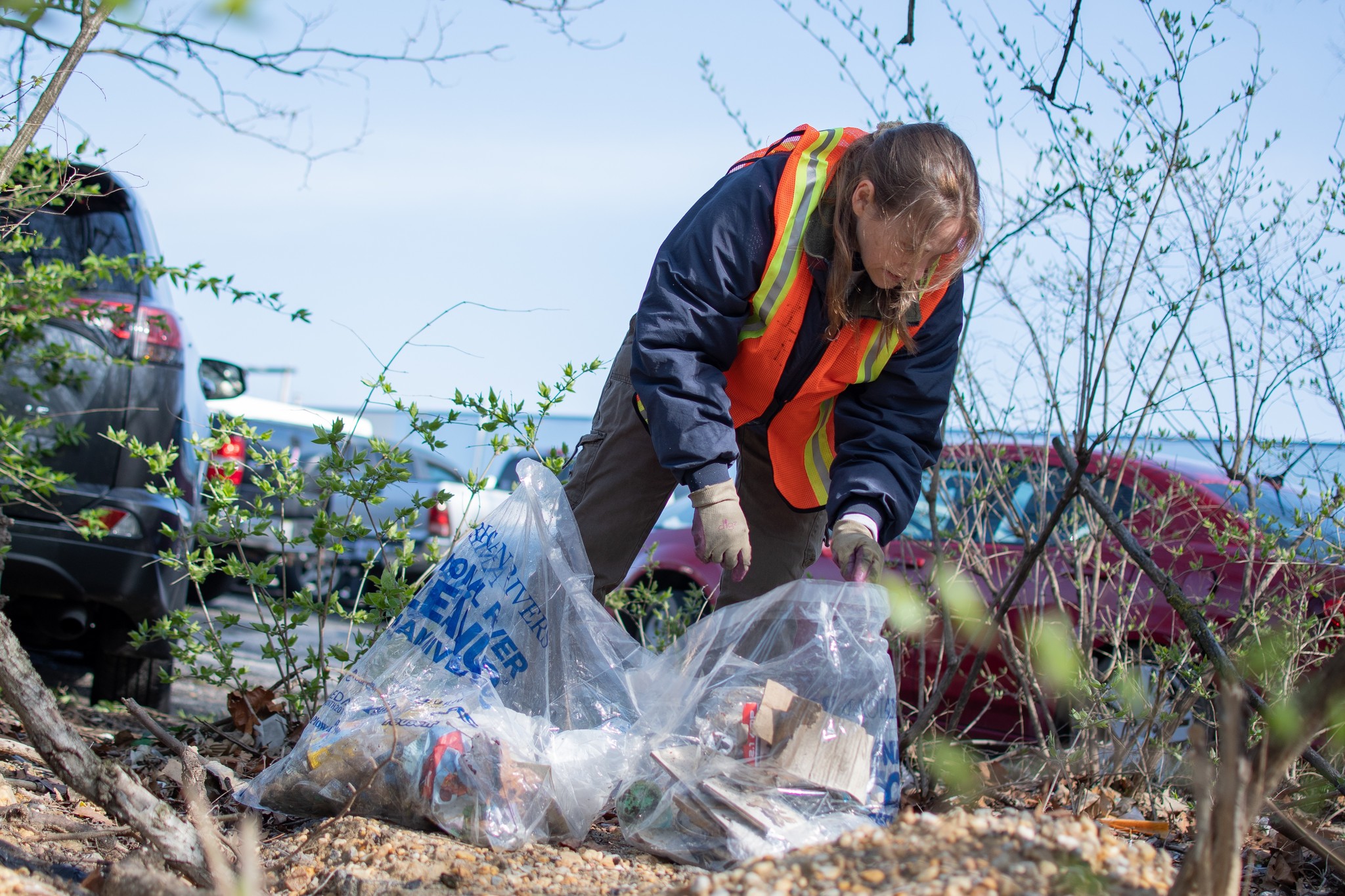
185 392 476 612
611 431 1345 766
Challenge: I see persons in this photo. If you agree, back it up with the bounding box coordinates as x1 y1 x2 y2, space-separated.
507 122 980 722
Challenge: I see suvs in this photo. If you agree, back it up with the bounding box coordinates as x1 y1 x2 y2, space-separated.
0 147 246 709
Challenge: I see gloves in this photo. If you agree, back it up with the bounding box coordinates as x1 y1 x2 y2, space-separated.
831 519 883 585
687 478 752 584
